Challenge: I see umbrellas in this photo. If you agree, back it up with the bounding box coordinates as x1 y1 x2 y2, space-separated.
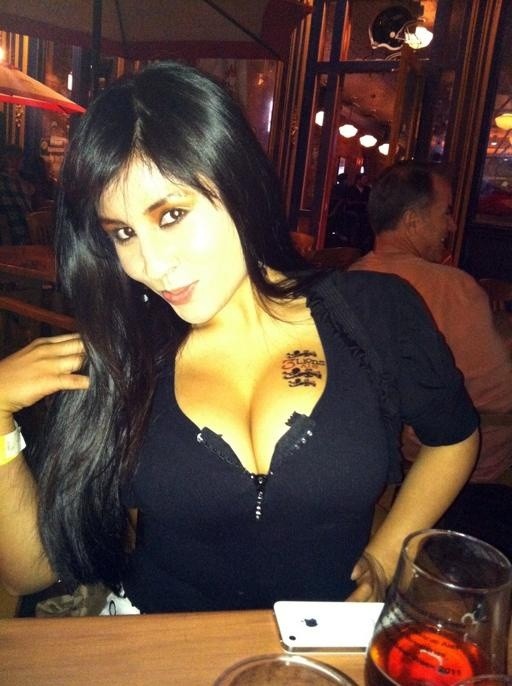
0 64 87 115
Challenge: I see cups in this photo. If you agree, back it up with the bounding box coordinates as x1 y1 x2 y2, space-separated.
359 523 512 686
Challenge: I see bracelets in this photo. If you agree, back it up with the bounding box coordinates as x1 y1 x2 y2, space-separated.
1 420 27 467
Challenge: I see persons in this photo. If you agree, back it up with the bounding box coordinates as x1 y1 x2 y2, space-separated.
1 142 38 246
343 157 511 601
0 60 481 617
343 172 373 251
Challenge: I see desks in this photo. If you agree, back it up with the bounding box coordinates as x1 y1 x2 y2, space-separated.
1 591 512 686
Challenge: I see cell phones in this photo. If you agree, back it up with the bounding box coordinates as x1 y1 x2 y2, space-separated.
271 600 384 655
